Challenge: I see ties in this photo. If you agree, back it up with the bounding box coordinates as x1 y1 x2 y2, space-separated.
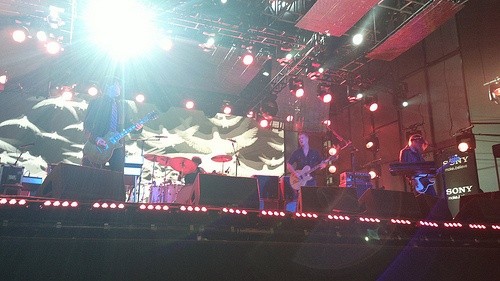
110 98 117 132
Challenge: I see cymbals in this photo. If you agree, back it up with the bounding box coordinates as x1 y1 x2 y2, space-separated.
169 157 197 174
144 153 159 162
156 155 171 167
211 154 232 163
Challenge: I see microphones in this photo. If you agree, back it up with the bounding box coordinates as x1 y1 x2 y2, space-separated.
230 139 236 142
21 142 36 147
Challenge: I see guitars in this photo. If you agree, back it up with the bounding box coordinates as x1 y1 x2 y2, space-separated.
415 155 460 194
83 111 160 166
288 152 341 191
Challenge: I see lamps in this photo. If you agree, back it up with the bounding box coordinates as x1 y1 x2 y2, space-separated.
489 77 500 104
0 10 407 178
456 126 476 153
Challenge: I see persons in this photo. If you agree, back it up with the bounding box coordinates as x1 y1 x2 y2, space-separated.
178 156 207 204
287 132 326 191
399 134 440 196
83 76 143 173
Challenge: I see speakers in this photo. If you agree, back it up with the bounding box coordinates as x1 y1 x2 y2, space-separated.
188 173 500 224
37 161 126 204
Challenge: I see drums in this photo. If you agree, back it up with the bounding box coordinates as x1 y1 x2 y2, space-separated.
175 185 192 204
148 183 185 204
